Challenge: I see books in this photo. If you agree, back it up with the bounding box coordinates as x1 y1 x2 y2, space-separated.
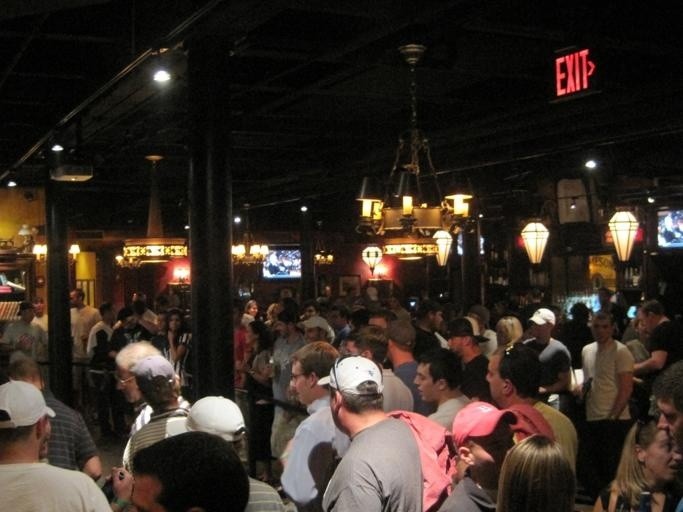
0 300 22 319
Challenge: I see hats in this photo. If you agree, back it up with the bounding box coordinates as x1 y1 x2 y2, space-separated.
452 402 517 451
529 309 556 325
17 303 33 314
470 306 491 328
317 357 384 396
0 378 56 428
382 320 416 347
186 397 247 444
298 316 335 342
448 322 490 343
134 356 175 397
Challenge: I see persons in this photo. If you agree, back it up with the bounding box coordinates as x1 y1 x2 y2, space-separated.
0 304 50 386
115 283 632 356
653 360 683 512
0 379 133 511
0 357 102 483
63 288 102 431
413 349 475 434
315 352 423 511
494 433 574 511
591 418 683 511
384 318 438 419
634 300 682 416
182 396 288 511
574 311 637 501
441 317 490 397
279 341 350 510
229 298 252 478
29 297 50 335
133 431 250 511
121 354 191 469
343 325 413 417
86 302 115 437
522 308 573 422
627 317 655 419
483 341 578 477
435 401 519 511
236 318 275 484
108 341 187 438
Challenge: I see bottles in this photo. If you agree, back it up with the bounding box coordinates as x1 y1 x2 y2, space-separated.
100 471 125 504
639 492 655 512
268 357 275 378
576 375 594 409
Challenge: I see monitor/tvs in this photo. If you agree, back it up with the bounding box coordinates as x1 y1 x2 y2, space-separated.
258 243 306 284
656 210 682 249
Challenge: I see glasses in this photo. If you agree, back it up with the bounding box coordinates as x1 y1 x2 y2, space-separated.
114 372 140 384
333 352 382 387
504 348 514 361
288 372 313 379
